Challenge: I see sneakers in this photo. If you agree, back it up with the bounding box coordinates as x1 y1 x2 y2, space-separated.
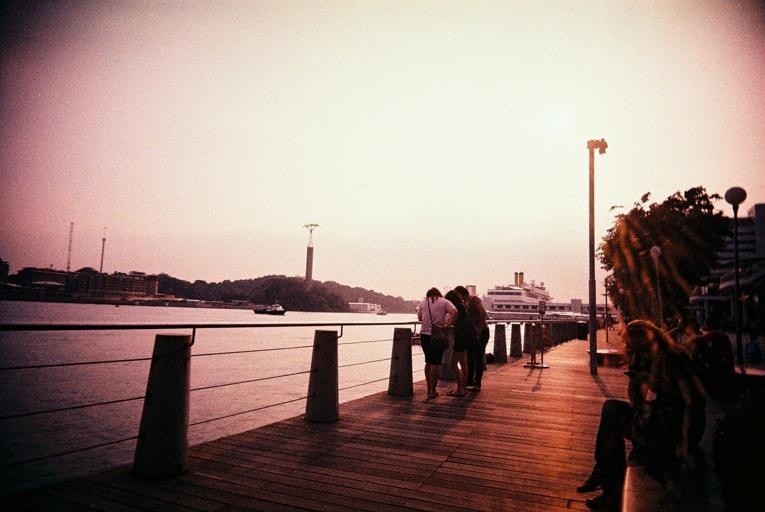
576 480 603 508
428 384 480 397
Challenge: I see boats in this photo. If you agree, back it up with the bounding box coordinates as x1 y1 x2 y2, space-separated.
377 307 388 316
480 271 617 323
253 301 289 317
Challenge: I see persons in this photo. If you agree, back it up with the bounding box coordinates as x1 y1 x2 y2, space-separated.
417 287 458 397
576 318 705 510
456 285 490 393
443 290 472 396
680 314 764 374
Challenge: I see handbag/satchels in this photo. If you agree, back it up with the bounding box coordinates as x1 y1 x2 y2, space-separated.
431 324 449 348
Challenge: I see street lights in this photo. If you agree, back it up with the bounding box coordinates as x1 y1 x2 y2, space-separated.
724 182 754 373
602 291 611 331
300 222 321 295
589 134 608 370
648 244 666 328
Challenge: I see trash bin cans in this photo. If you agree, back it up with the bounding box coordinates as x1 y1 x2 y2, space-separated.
576 321 588 340
